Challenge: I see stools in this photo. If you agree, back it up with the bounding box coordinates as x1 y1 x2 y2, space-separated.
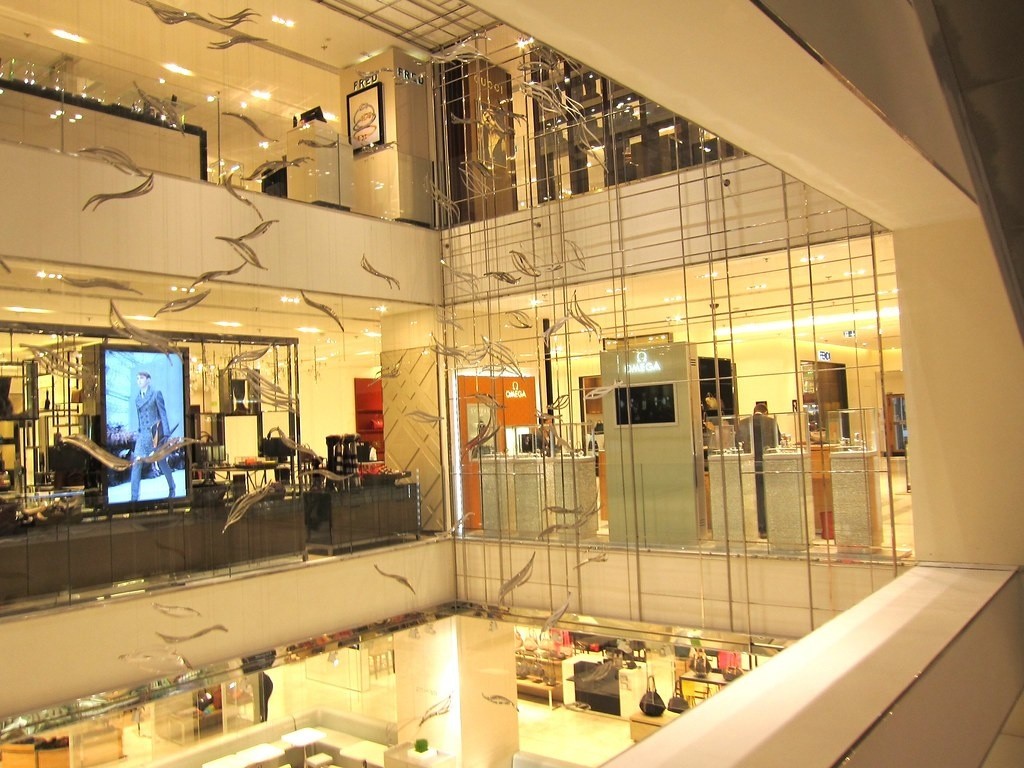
306 751 333 768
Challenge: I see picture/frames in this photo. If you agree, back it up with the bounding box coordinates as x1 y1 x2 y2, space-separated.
345 81 384 154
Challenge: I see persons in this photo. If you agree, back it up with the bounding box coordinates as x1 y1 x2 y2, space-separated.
255 671 274 722
537 413 555 452
129 370 176 502
735 404 781 539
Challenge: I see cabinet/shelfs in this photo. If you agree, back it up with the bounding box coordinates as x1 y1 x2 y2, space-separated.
514 646 571 702
0 320 307 607
630 708 681 743
301 482 421 555
285 119 353 211
679 670 730 697
0 78 207 181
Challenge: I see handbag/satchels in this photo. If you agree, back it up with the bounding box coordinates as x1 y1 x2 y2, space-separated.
47 442 87 489
723 652 743 682
668 681 689 714
191 474 229 508
689 648 711 677
639 676 666 716
513 627 566 686
260 426 291 457
213 685 223 710
196 689 210 711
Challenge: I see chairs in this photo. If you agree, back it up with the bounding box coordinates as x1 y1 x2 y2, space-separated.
367 635 391 680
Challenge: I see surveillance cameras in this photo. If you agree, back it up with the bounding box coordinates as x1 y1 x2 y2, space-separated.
710 304 719 308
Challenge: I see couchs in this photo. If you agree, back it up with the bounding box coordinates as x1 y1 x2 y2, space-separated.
143 704 401 768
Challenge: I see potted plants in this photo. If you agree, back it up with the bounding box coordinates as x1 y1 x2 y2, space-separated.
406 739 437 760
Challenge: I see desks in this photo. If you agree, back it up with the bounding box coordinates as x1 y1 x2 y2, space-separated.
339 739 390 767
201 741 285 768
282 727 326 768
